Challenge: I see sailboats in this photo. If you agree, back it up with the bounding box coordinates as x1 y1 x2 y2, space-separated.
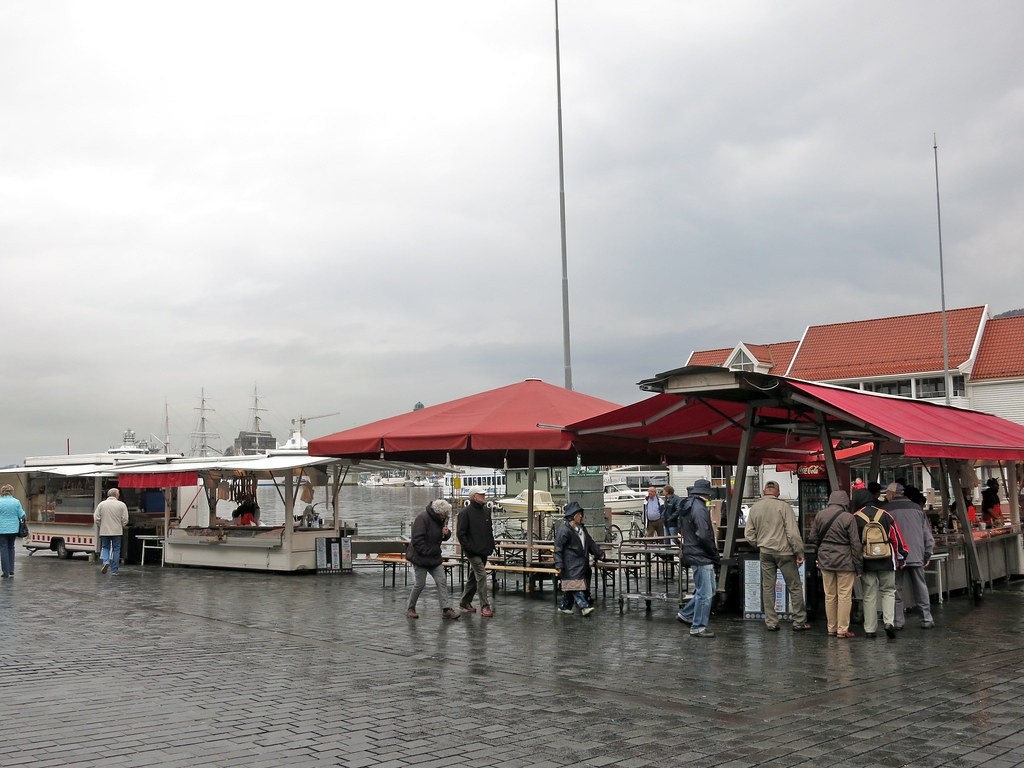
185 386 226 482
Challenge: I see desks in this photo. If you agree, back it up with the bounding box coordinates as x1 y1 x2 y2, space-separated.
399 536 681 586
136 536 165 567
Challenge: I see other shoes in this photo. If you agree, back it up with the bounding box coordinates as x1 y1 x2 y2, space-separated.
767 626 780 631
1 573 8 577
793 624 811 631
836 632 854 637
102 564 109 573
827 632 836 635
582 607 594 616
866 632 877 638
558 607 574 614
884 623 895 639
112 573 119 575
921 621 935 629
10 571 14 576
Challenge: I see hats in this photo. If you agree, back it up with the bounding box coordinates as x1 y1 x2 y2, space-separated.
689 479 715 494
880 483 904 493
564 501 584 517
987 477 999 486
469 485 487 497
765 480 779 490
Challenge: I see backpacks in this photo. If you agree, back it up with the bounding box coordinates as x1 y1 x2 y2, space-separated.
855 509 892 559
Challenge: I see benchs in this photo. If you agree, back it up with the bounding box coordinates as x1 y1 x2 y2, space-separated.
485 565 560 607
603 558 679 579
378 558 462 595
589 563 652 597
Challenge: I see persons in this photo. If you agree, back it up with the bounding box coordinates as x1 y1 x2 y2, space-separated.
808 490 863 639
880 483 936 630
981 477 1008 529
678 479 722 638
0 484 28 578
642 487 665 544
405 499 460 619
553 501 605 617
94 488 129 576
662 485 682 550
456 486 495 617
744 481 811 632
850 477 980 541
232 494 260 527
852 491 909 640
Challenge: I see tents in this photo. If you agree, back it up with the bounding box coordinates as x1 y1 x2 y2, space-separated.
309 379 626 607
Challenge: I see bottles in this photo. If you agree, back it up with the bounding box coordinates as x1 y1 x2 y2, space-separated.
807 498 818 512
925 505 939 529
805 559 822 578
805 515 814 527
939 521 948 534
806 530 818 544
818 498 829 511
805 483 817 497
819 483 828 497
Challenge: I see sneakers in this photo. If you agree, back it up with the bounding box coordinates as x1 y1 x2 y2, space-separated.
405 608 419 618
442 609 460 619
690 629 714 637
676 615 692 627
480 607 493 617
459 602 476 611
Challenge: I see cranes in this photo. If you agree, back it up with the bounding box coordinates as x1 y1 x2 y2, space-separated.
292 413 343 432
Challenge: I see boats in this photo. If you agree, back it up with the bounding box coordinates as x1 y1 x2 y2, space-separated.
495 489 558 514
440 466 506 502
354 469 440 487
598 482 647 515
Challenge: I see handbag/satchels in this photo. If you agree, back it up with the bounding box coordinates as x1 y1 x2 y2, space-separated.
17 516 28 537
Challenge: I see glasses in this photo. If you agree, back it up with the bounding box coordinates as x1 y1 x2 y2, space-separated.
476 493 485 496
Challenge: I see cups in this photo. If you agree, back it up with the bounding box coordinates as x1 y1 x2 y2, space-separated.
952 520 959 532
979 522 986 530
995 518 1001 528
